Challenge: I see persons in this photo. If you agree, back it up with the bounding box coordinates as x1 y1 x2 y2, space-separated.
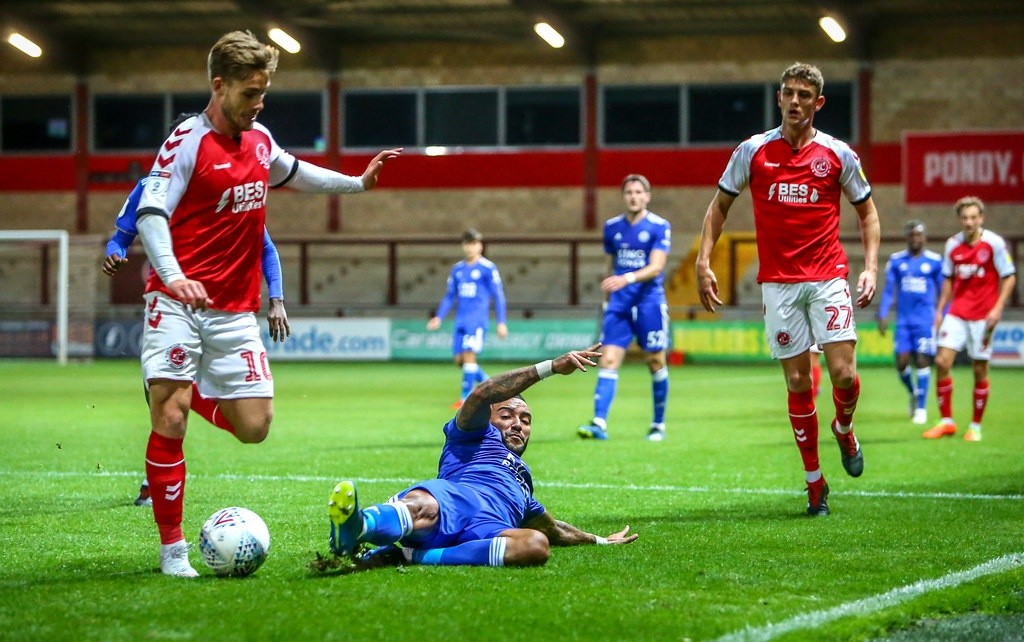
426 227 509 406
308 343 640 572
577 174 671 441
877 195 1017 440
695 61 880 518
103 31 404 578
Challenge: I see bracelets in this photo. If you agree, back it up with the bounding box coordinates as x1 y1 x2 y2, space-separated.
536 359 553 378
592 533 607 545
622 272 636 283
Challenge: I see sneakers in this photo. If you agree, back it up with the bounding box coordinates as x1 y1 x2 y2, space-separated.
160 546 202 580
329 481 362 558
912 408 928 425
576 423 608 440
646 428 666 445
831 418 864 476
355 542 404 567
135 478 154 505
965 428 982 442
923 422 957 438
805 483 830 516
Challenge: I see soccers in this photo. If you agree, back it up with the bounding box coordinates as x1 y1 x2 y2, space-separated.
199 506 272 578
